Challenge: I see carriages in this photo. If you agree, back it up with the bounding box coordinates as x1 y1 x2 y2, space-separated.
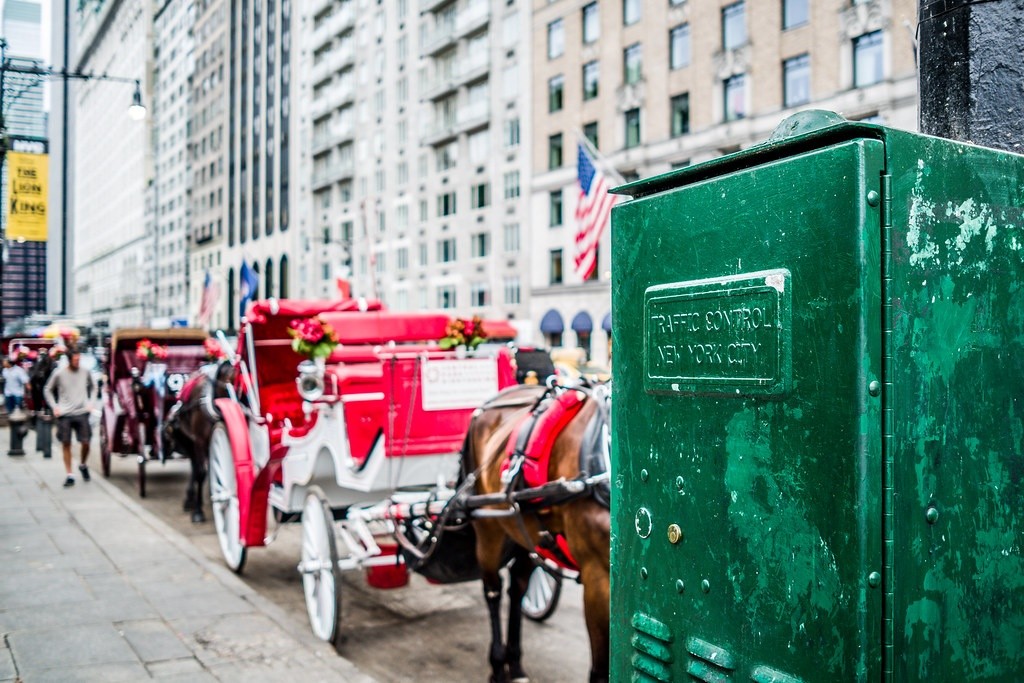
100 326 249 524
207 280 609 683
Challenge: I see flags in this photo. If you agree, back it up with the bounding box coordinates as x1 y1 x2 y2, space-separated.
200 273 211 321
572 142 620 283
240 258 257 319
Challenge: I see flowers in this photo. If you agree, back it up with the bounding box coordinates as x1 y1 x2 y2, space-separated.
61 330 80 347
135 339 168 359
438 316 487 356
288 320 336 359
205 339 226 362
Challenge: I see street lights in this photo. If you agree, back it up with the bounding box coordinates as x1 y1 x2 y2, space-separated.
0 39 147 241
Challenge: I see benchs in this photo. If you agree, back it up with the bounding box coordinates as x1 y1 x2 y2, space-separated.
244 300 380 436
113 336 212 415
313 314 455 452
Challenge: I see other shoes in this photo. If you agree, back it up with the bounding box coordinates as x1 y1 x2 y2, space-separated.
63 477 75 487
78 465 90 481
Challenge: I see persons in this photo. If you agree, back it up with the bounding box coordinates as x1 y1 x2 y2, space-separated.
43 350 95 488
3 341 111 428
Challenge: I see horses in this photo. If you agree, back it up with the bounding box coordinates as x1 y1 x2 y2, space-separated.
453 382 611 683
161 358 251 525
